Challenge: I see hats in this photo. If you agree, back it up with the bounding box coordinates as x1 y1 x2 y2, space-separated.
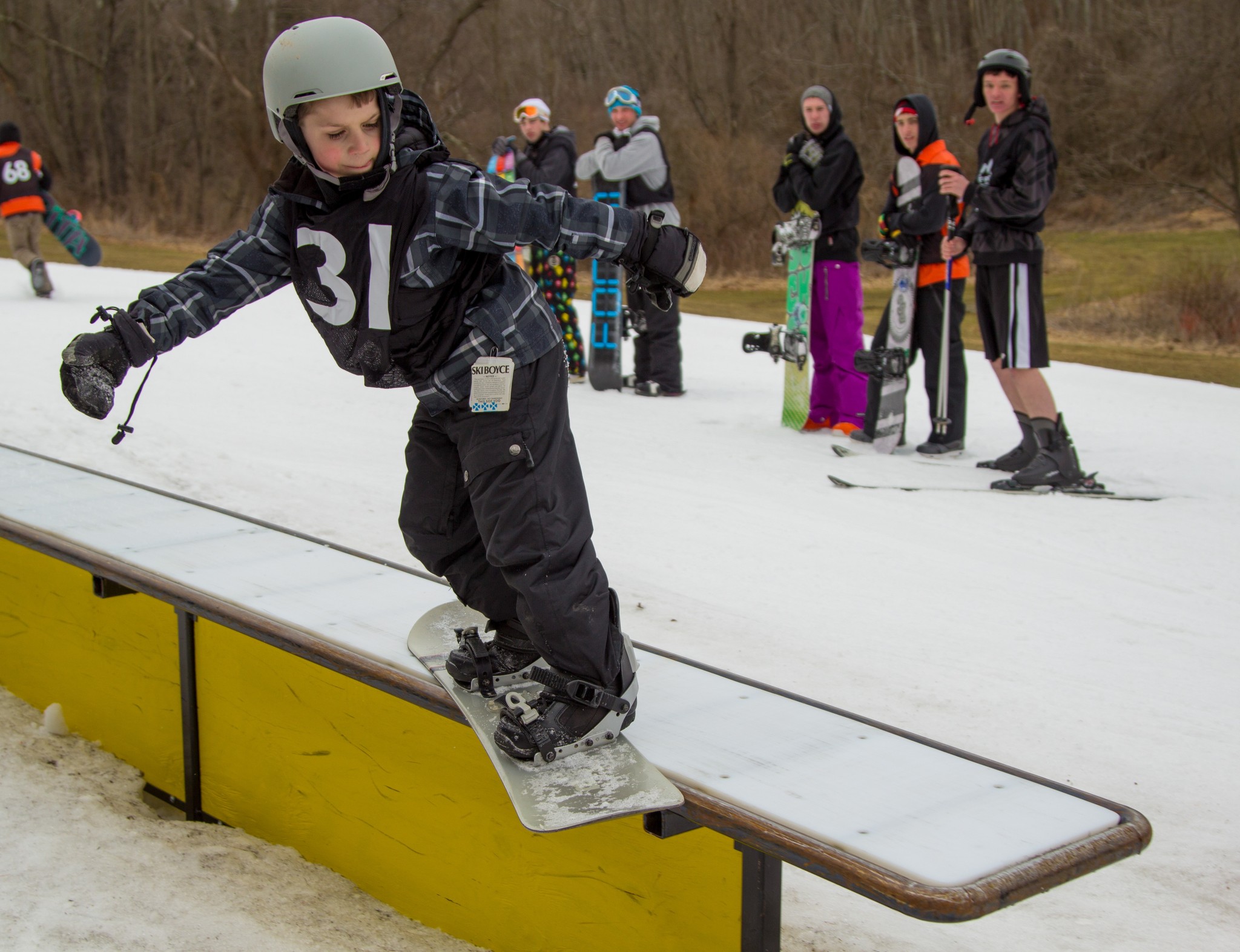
801 85 833 114
894 98 918 120
513 98 550 123
0 121 21 145
604 85 642 117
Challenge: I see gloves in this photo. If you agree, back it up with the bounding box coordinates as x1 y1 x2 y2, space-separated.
58 307 156 445
786 133 806 157
877 213 889 242
889 229 919 250
491 135 516 155
799 140 824 167
612 135 631 151
623 210 707 313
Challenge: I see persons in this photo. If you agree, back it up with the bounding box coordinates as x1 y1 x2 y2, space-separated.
939 47 1085 495
886 97 974 457
491 96 589 384
575 84 686 398
0 121 55 299
59 15 710 760
772 86 870 437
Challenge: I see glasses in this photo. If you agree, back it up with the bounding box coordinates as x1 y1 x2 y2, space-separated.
605 86 641 107
516 106 537 119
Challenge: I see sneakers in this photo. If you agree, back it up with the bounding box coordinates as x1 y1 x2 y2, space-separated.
31 257 45 289
495 648 637 759
446 621 543 686
916 432 965 458
568 374 585 384
832 421 861 438
849 428 906 448
799 415 832 433
622 374 650 389
635 380 684 396
36 290 51 298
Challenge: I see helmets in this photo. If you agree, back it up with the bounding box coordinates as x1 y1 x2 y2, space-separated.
963 49 1032 126
262 15 403 193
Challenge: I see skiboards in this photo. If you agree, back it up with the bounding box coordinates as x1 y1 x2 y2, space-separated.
828 442 1170 501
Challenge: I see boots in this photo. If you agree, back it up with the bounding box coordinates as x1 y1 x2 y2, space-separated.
1012 412 1080 487
994 411 1039 468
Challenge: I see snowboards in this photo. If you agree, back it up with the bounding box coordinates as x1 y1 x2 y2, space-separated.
592 133 627 392
35 187 101 269
851 154 929 460
742 130 826 430
407 603 686 836
484 148 534 280
